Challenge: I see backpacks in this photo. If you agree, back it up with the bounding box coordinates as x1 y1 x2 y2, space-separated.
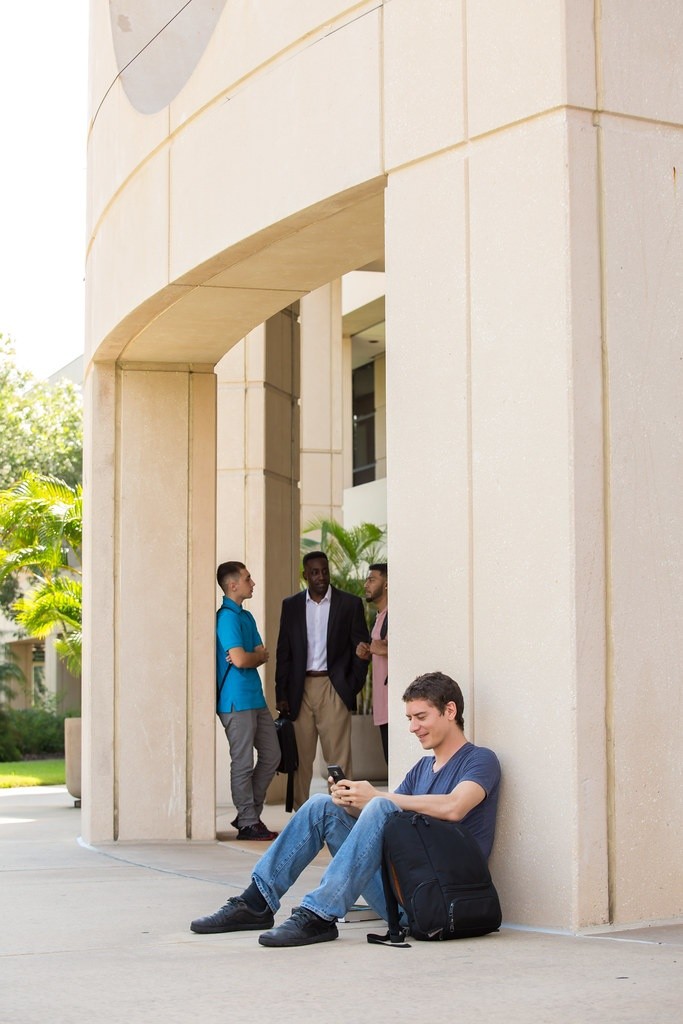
380 810 504 948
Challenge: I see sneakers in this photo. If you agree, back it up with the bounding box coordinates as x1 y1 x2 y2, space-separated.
236 821 278 840
231 815 240 830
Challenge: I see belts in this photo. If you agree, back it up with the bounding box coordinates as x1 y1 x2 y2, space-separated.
305 671 328 677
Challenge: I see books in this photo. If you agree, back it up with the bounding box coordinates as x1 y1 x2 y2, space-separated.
292 904 383 923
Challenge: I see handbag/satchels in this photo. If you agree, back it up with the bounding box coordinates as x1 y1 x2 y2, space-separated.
271 710 300 774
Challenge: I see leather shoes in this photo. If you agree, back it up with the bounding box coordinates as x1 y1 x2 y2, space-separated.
258 907 340 947
189 895 274 932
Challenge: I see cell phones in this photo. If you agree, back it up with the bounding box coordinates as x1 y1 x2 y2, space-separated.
327 765 350 789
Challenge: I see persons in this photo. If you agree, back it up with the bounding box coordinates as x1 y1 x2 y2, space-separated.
215 560 282 841
274 551 370 814
191 672 500 948
355 562 388 764
